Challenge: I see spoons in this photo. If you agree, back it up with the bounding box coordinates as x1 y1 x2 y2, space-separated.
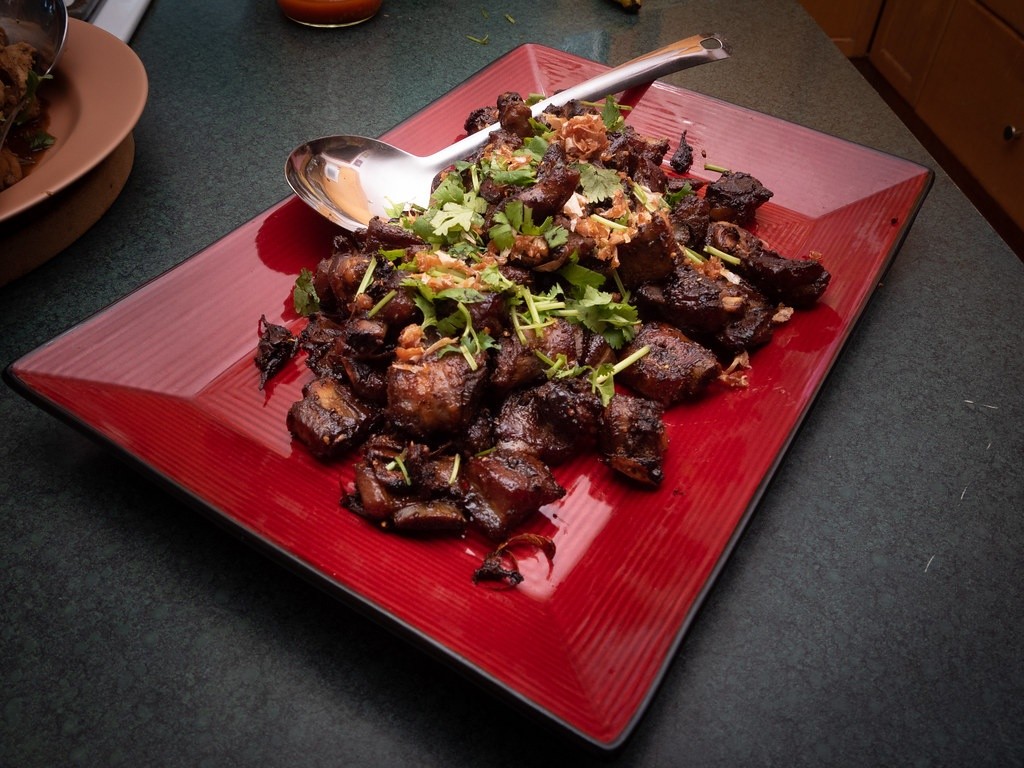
283 31 734 233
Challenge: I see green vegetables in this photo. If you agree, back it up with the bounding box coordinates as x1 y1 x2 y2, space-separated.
293 89 742 405
0 72 57 151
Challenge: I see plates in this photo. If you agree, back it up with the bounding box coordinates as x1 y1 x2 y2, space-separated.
0 41 935 749
0 18 148 222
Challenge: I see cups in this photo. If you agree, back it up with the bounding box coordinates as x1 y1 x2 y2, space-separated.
278 0 381 29
